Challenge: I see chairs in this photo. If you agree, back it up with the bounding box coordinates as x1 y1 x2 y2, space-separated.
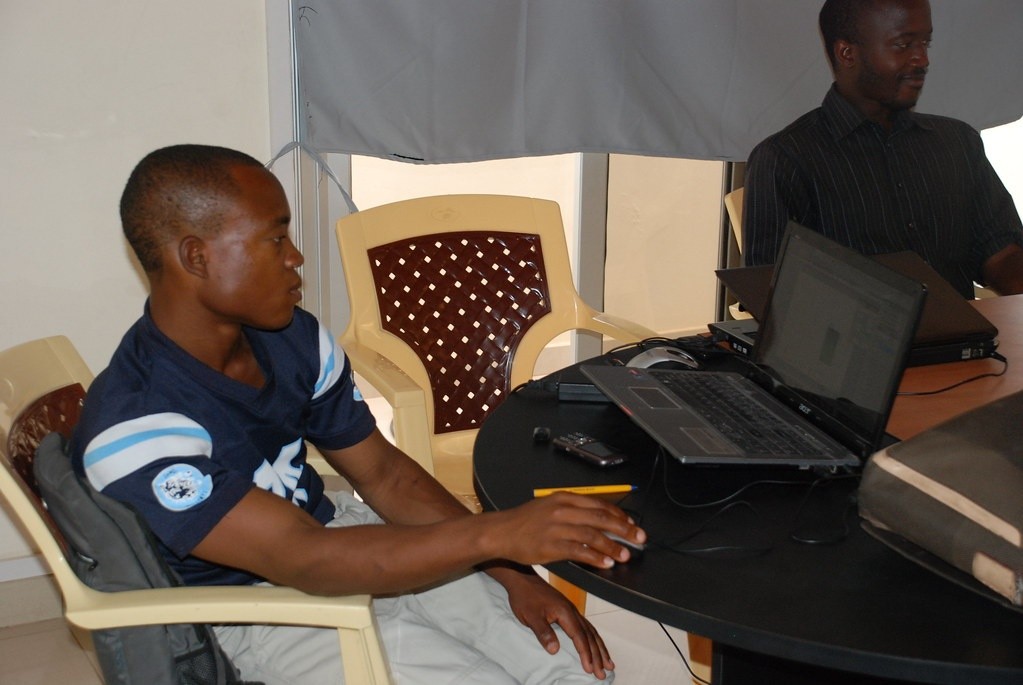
723 187 1000 320
335 194 662 617
0 335 392 685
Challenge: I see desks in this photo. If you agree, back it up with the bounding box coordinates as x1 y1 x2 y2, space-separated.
472 292 1023 685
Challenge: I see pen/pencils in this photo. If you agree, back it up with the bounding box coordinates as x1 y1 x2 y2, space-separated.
533 484 638 498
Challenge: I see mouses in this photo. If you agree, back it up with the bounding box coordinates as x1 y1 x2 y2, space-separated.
582 531 646 562
625 346 704 372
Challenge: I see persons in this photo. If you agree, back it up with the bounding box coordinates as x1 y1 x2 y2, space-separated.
741 0 1023 301
70 145 646 685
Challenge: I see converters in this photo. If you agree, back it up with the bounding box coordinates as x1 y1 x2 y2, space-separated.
557 374 612 402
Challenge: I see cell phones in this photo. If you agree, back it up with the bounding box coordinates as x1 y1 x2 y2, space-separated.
553 432 629 466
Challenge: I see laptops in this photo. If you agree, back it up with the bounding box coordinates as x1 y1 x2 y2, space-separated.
578 220 928 478
707 249 999 369
